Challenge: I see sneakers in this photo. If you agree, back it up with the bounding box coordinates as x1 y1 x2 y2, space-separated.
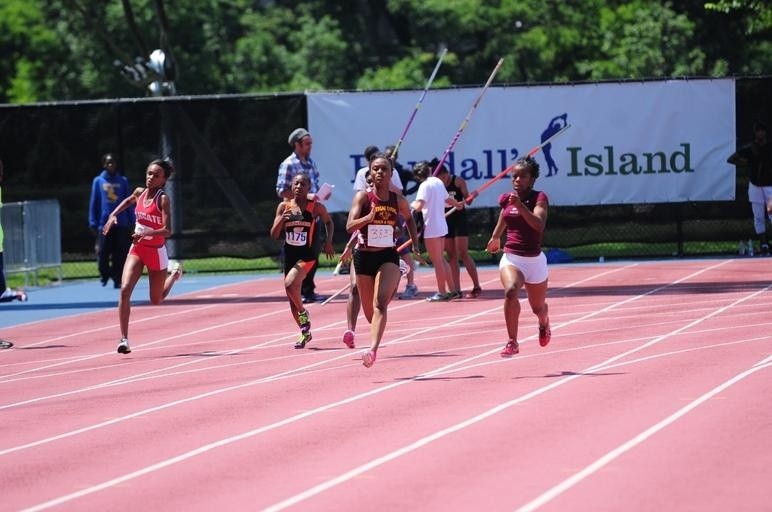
500 338 519 357
298 308 311 333
172 263 182 281
343 330 355 349
398 283 419 299
294 330 312 348
362 351 376 367
539 315 551 347
117 338 131 354
426 288 481 302
301 295 326 303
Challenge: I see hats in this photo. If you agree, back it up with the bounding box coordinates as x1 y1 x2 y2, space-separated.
288 128 310 148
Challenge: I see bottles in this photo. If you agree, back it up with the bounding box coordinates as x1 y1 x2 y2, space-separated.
747 239 754 257
599 256 605 263
738 240 746 256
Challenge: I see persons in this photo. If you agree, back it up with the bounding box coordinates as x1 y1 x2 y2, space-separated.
270 174 335 349
430 157 482 299
410 162 463 302
346 153 426 368
486 155 551 357
337 145 420 348
0 186 28 303
727 120 772 257
276 128 333 304
88 154 135 287
102 157 183 354
